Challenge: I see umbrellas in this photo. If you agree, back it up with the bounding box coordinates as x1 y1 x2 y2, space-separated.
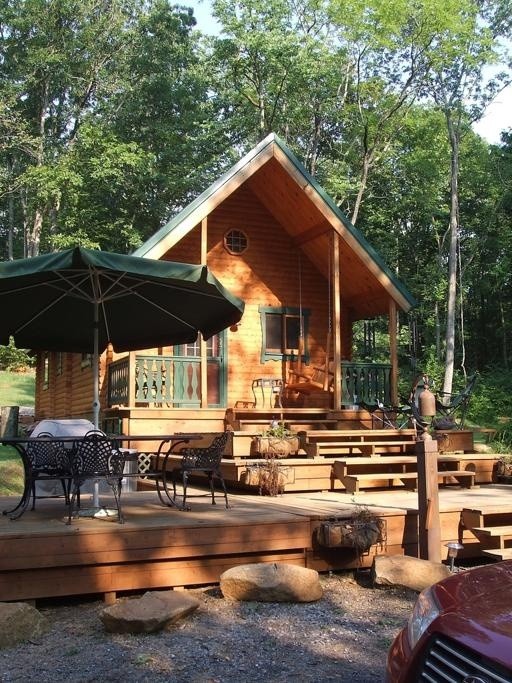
0 246 246 506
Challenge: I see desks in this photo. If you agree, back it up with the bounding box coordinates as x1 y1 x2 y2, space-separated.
1 434 204 520
252 378 285 408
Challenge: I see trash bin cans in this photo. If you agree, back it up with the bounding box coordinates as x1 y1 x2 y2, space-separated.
116 448 138 494
1 406 19 446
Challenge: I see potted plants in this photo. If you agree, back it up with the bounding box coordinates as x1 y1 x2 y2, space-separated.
253 422 301 459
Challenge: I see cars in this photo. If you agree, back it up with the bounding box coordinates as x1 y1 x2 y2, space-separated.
386 560 512 683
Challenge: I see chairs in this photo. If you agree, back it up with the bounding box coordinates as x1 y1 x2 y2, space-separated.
353 373 477 430
171 433 229 512
26 429 126 526
136 359 171 407
